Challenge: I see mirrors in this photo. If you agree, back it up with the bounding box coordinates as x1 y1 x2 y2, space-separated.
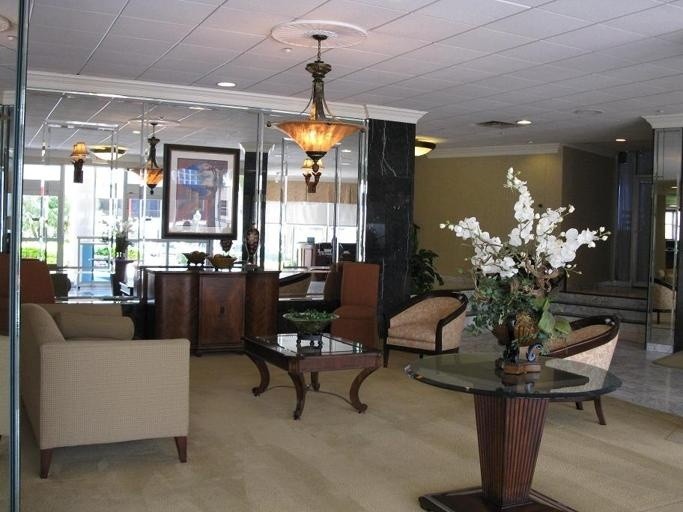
637 124 682 356
19 85 371 307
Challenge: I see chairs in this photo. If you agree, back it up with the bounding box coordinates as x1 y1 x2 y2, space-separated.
328 258 388 354
382 287 469 371
648 274 678 327
275 270 313 300
535 314 624 429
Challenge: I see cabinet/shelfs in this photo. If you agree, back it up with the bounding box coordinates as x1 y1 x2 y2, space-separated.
136 260 284 357
129 263 217 301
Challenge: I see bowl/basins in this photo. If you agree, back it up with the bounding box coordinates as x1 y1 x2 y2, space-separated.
207 257 237 269
183 253 209 263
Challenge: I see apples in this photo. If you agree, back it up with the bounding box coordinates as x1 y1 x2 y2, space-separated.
199 252 206 257
192 251 199 257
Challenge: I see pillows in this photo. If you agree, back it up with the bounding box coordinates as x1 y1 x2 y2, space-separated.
46 302 140 340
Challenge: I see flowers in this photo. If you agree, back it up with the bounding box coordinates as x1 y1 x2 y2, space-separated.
96 213 139 248
435 163 614 347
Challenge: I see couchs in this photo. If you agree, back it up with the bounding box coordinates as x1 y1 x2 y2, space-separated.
15 298 195 479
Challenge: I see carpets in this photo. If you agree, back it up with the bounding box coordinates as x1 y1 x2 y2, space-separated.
11 327 683 511
649 349 682 371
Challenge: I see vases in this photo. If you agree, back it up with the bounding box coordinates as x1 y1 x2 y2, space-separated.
491 288 546 381
112 233 130 260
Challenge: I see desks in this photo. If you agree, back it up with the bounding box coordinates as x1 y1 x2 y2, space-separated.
403 354 626 511
85 253 143 301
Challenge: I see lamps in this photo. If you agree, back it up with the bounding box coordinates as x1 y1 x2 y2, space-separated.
128 115 178 198
262 19 370 172
66 140 89 183
414 136 438 160
299 154 325 195
84 141 129 164
